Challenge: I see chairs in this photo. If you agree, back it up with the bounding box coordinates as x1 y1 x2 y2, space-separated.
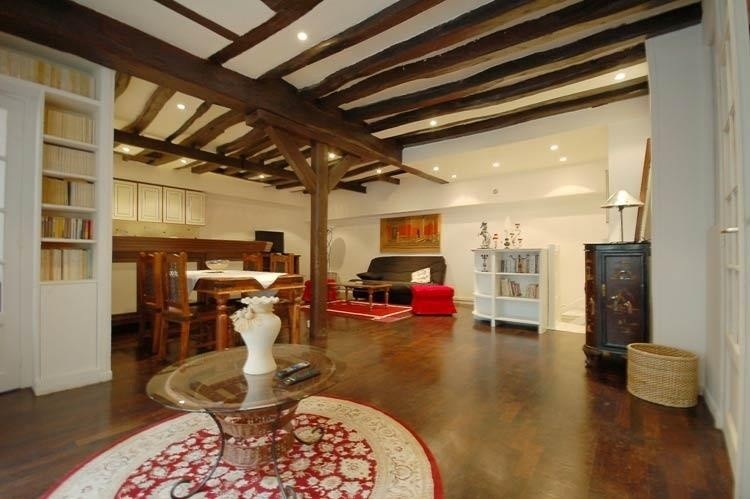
134 250 307 366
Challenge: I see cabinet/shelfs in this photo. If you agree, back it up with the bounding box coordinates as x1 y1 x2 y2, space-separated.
580 240 652 370
113 179 163 224
163 187 206 226
33 90 116 397
0 27 116 105
470 244 549 334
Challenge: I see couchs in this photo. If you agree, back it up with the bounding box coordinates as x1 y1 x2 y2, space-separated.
350 255 447 306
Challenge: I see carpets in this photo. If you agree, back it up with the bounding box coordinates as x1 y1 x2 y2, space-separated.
302 298 414 324
42 390 445 498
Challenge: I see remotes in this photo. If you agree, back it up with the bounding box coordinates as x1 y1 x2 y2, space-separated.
282 369 321 386
277 360 311 378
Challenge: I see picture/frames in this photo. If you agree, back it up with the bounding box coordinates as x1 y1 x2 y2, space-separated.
378 214 442 255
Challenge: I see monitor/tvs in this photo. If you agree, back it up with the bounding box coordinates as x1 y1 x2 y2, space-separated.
254 230 285 253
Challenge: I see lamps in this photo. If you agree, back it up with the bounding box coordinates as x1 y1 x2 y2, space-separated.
599 188 646 244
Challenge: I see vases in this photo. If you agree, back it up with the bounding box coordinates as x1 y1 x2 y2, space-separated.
240 370 277 409
227 294 283 376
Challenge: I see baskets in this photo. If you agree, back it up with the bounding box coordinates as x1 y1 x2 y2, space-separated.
626 343 698 408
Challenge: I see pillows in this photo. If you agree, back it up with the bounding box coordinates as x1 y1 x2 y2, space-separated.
410 266 432 285
356 272 384 280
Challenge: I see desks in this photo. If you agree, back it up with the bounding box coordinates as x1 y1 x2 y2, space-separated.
143 342 347 498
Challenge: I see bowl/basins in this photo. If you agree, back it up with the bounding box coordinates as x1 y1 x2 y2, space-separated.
205 259 230 272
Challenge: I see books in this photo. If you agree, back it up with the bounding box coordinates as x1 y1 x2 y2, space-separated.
0 45 97 102
39 109 97 281
499 254 540 299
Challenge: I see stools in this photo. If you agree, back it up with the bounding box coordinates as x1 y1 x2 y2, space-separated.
304 278 338 304
411 284 458 316
